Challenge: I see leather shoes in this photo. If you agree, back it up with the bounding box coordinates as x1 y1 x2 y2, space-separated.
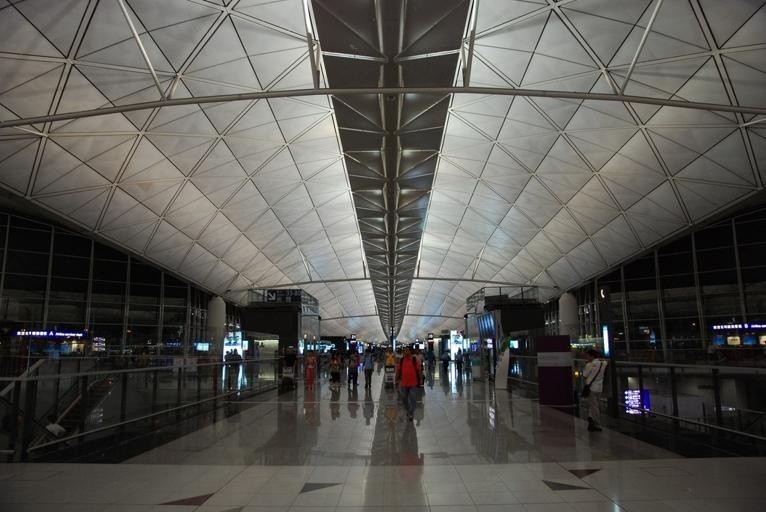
586 416 603 432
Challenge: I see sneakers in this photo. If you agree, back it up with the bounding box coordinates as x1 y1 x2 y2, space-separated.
406 412 413 421
365 385 371 388
348 383 359 386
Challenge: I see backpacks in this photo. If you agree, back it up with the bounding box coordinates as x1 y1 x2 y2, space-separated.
400 355 426 388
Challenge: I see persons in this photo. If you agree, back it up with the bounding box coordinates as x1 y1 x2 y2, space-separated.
583 348 610 431
224 349 242 388
284 344 470 421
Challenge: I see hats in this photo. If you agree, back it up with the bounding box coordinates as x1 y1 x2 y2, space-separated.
585 349 599 357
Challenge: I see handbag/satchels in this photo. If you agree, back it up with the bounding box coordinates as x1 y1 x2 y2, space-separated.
579 385 591 399
